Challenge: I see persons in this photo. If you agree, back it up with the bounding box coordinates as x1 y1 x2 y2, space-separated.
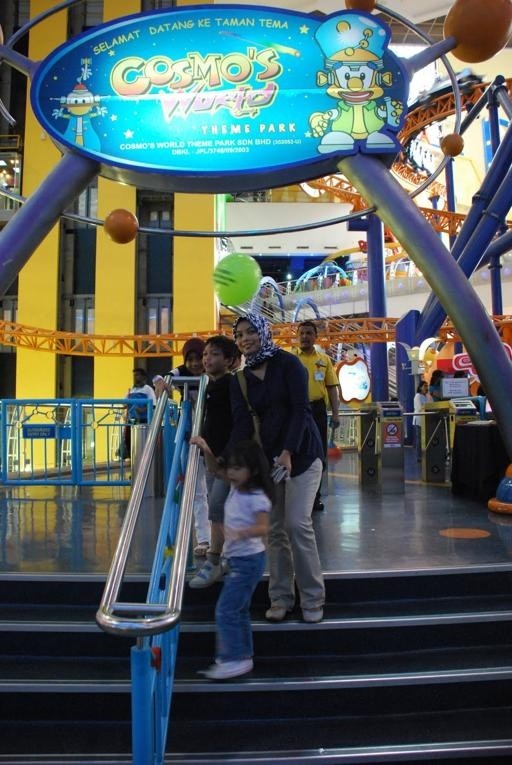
187 435 272 679
115 301 343 624
412 379 434 462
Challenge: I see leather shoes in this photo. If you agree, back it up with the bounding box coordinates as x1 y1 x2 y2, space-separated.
302 606 324 622
313 499 325 510
266 607 287 621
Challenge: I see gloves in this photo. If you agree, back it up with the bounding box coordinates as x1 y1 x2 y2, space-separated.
270 456 292 485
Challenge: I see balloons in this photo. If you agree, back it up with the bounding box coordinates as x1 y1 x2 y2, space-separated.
213 252 263 305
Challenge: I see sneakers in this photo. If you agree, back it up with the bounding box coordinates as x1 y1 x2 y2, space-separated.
189 560 221 588
204 659 254 680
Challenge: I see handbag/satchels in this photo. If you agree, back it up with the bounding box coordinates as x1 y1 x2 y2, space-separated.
236 370 272 473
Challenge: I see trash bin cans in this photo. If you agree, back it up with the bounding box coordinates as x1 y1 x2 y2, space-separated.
131 424 162 497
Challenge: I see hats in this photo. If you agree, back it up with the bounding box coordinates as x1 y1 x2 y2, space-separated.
183 338 204 366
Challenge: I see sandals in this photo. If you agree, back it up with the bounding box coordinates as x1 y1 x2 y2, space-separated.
193 542 210 555
